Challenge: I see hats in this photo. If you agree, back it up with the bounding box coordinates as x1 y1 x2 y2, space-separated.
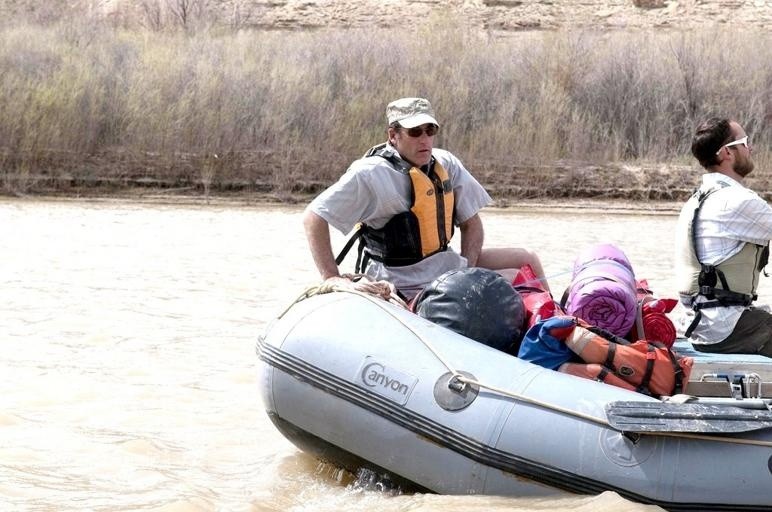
386 97 440 128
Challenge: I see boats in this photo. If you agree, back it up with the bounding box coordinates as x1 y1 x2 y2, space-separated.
256 282 772 512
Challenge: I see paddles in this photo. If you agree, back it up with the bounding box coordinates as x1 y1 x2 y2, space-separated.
605 401 771 433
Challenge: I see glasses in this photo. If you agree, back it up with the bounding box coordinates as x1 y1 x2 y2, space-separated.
717 135 749 156
388 125 437 137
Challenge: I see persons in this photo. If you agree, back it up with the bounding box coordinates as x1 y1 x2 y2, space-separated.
304 97 553 310
674 117 772 358
